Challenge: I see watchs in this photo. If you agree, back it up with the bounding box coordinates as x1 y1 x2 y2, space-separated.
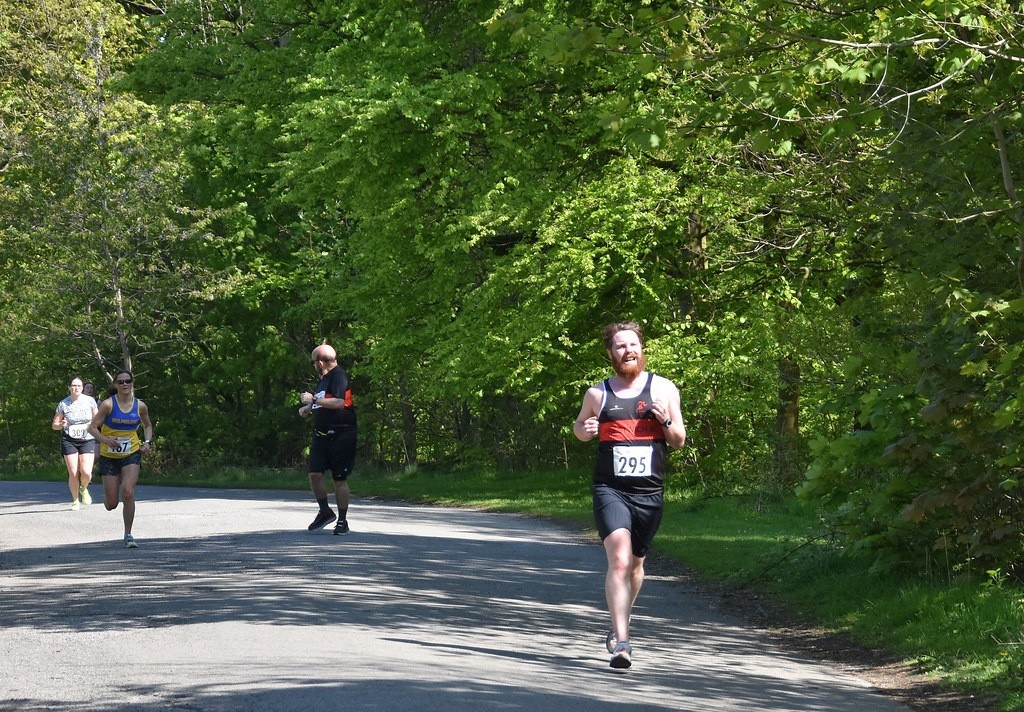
312 396 318 404
145 439 153 447
660 418 672 427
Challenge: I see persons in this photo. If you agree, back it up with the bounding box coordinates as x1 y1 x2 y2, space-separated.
298 345 357 536
52 370 152 548
573 322 686 668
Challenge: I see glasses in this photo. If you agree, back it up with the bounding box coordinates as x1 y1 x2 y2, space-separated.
116 379 132 384
311 360 324 365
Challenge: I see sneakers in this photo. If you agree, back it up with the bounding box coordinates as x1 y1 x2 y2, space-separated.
79 486 92 505
331 519 349 535
610 642 632 668
72 498 80 510
124 534 138 548
104 497 112 511
308 507 336 532
607 615 631 652
